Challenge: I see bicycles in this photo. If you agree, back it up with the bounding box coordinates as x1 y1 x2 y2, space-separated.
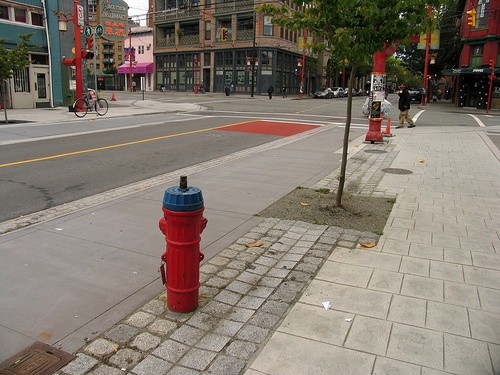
75 89 109 118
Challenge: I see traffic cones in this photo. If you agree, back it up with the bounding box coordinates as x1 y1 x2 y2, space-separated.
384 117 394 137
110 93 116 101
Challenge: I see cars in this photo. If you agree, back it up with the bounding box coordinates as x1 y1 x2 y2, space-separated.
355 88 363 96
331 87 345 98
342 87 356 97
313 87 334 99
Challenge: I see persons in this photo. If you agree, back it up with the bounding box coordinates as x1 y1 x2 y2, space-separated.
282 83 288 98
433 89 443 101
268 84 274 100
397 83 416 129
133 80 136 91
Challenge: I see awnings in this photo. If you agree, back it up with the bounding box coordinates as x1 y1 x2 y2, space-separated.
440 67 492 75
119 63 153 73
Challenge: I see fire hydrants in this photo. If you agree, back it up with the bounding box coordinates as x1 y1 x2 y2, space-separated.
158 173 208 314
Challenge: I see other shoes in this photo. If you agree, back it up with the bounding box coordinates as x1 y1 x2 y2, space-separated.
395 126 403 128
408 125 415 128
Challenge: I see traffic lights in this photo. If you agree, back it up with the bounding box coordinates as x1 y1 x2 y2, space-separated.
220 27 228 42
466 7 476 27
